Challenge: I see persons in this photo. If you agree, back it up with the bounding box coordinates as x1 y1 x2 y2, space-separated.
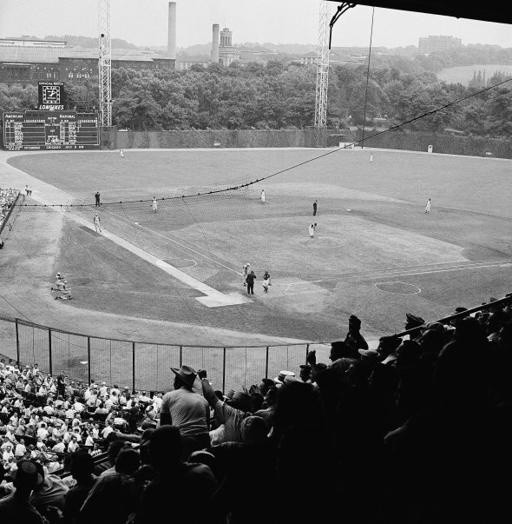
312 199 319 216
50 272 73 300
152 196 157 214
424 198 433 215
261 270 271 294
243 262 250 286
25 183 33 196
309 222 319 238
94 192 101 207
93 213 103 234
246 270 256 294
0 295 511 524
260 189 266 203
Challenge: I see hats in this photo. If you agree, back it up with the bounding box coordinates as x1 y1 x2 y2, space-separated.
171 366 198 386
274 371 296 384
